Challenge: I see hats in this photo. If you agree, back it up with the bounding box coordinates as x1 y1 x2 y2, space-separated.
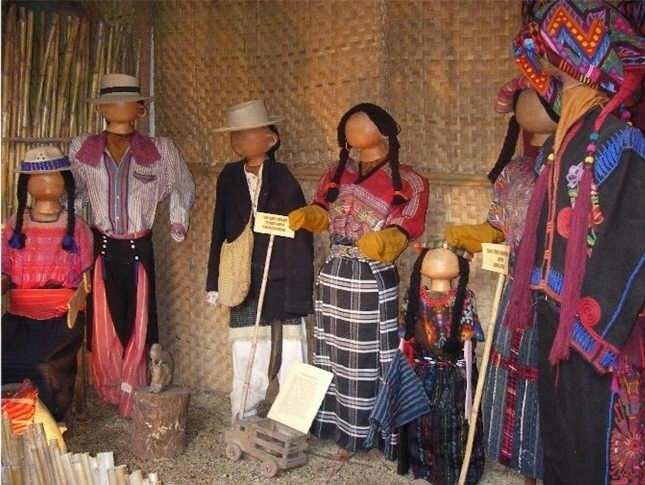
210 100 287 134
13 146 82 174
86 72 153 105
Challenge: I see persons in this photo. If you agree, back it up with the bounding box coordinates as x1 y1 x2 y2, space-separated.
204 97 315 430
1 143 96 432
58 73 196 418
509 0 645 484
397 230 487 484
288 101 433 462
442 66 568 485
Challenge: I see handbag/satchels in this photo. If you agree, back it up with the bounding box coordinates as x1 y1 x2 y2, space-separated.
216 224 254 307
